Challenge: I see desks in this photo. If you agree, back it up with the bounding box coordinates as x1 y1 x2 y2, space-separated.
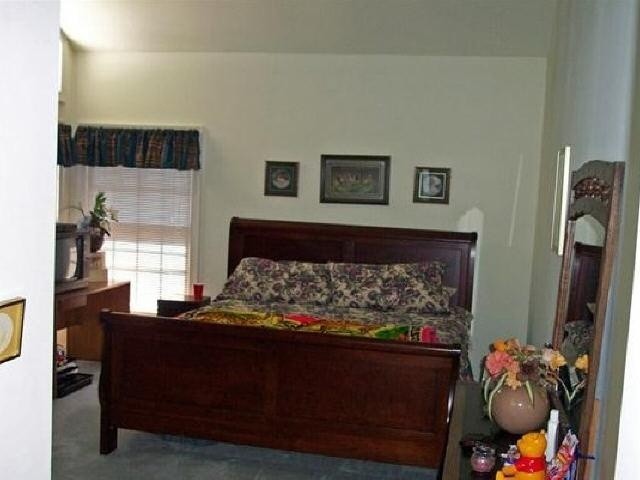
55 281 131 363
440 380 521 480
156 295 211 317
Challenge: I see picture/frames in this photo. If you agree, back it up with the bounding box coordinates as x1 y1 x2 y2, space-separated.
0 296 27 365
548 146 571 255
319 154 390 205
412 166 449 205
263 160 298 197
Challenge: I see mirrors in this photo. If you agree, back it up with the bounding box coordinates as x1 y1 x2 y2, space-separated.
549 159 628 449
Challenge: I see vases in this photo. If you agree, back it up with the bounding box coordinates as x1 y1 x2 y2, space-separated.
487 384 550 435
81 227 105 252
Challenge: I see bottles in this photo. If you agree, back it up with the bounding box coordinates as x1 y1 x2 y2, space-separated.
544 409 560 463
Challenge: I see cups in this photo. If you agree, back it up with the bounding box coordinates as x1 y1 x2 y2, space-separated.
194 283 203 300
471 446 496 473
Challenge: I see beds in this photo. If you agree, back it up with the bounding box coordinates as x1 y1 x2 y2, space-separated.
96 216 477 469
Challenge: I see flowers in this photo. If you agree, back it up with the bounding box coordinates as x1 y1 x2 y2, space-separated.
72 191 119 237
478 337 573 421
572 348 593 401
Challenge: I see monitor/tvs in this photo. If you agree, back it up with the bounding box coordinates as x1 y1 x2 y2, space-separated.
55 228 90 294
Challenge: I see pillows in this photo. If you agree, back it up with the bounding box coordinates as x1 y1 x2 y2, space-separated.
208 257 455 312
